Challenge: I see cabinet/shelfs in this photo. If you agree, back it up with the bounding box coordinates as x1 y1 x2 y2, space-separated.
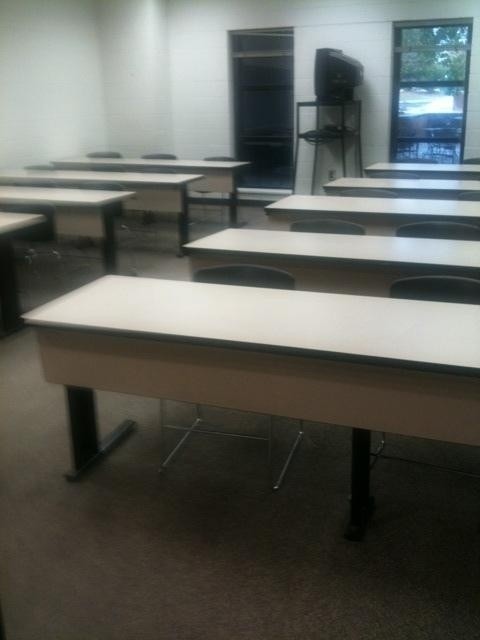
292 99 363 194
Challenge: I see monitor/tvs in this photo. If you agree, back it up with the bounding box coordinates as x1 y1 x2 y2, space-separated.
313 48 364 104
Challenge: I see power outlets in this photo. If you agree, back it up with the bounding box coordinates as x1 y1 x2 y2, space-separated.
328 169 336 180
